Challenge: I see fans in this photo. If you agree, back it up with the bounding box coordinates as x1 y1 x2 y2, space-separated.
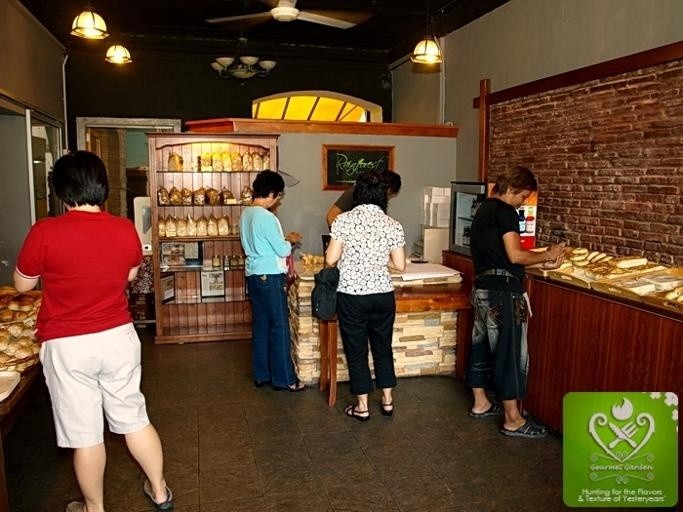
203 0 376 33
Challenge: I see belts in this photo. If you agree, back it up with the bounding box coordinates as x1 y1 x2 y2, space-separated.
475 268 521 280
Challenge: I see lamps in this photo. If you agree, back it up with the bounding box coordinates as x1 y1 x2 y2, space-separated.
406 0 443 65
67 0 112 42
209 22 277 88
102 22 134 66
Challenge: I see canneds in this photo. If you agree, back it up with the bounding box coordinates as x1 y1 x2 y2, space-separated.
212 254 245 266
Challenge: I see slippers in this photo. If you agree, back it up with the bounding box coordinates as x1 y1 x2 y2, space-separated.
143 478 173 510
468 399 505 419
66 500 102 512
499 419 549 439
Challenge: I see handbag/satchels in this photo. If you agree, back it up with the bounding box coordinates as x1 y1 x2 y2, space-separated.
311 253 339 321
512 293 530 325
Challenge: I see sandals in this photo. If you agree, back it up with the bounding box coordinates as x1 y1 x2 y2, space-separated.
253 379 307 392
381 401 394 417
344 404 370 422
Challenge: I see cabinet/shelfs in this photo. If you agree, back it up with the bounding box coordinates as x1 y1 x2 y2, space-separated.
416 222 449 268
141 129 280 346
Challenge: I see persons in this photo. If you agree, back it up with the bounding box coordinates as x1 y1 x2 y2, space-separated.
239 170 306 392
326 172 406 421
326 169 402 226
467 165 565 438
13 151 174 512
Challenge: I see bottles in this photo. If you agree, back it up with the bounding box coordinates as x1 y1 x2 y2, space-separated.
461 225 471 246
516 207 534 233
469 196 476 218
213 253 246 266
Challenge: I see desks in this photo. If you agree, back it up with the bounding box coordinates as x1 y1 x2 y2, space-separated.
309 281 478 410
0 352 46 511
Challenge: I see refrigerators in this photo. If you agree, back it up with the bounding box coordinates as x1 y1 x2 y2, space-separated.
446 179 538 257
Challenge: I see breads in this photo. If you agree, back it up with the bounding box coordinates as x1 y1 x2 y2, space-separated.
560 247 683 306
0 286 43 371
